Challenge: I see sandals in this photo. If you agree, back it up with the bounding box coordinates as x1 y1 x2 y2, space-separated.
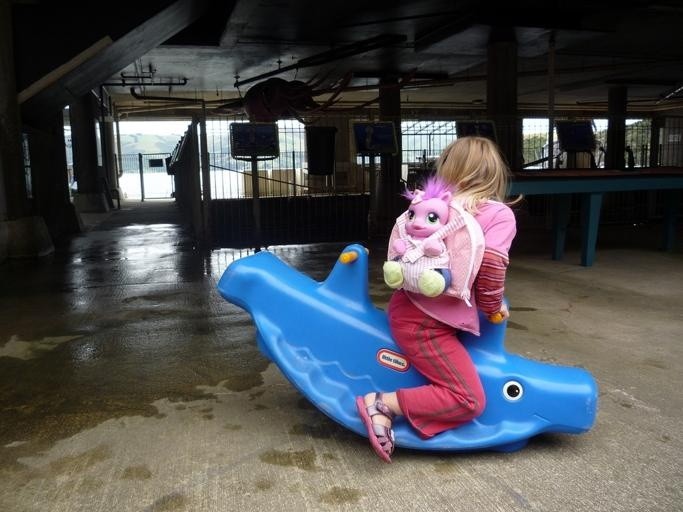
355 391 397 464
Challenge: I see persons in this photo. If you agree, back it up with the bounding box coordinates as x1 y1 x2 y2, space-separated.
351 136 521 466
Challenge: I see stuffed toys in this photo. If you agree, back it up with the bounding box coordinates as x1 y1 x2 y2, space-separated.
381 177 454 298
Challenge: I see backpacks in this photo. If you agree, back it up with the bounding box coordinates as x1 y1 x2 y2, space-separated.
383 189 486 308
